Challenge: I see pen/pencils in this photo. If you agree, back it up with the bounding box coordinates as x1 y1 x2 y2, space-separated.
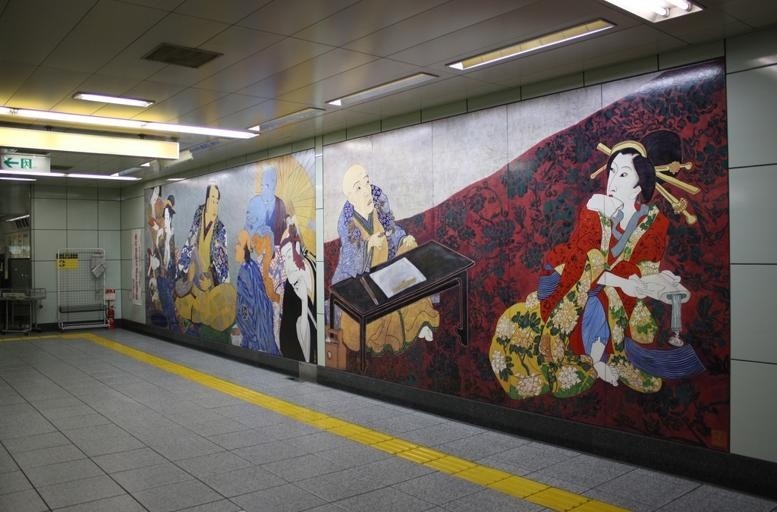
360 276 379 306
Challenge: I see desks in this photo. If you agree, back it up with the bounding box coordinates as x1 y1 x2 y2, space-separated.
329 239 475 371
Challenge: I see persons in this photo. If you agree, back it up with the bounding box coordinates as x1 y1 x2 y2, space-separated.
537 130 705 381
148 165 316 365
324 165 440 354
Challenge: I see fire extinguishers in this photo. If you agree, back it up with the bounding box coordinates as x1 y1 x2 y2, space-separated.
105 305 115 329
